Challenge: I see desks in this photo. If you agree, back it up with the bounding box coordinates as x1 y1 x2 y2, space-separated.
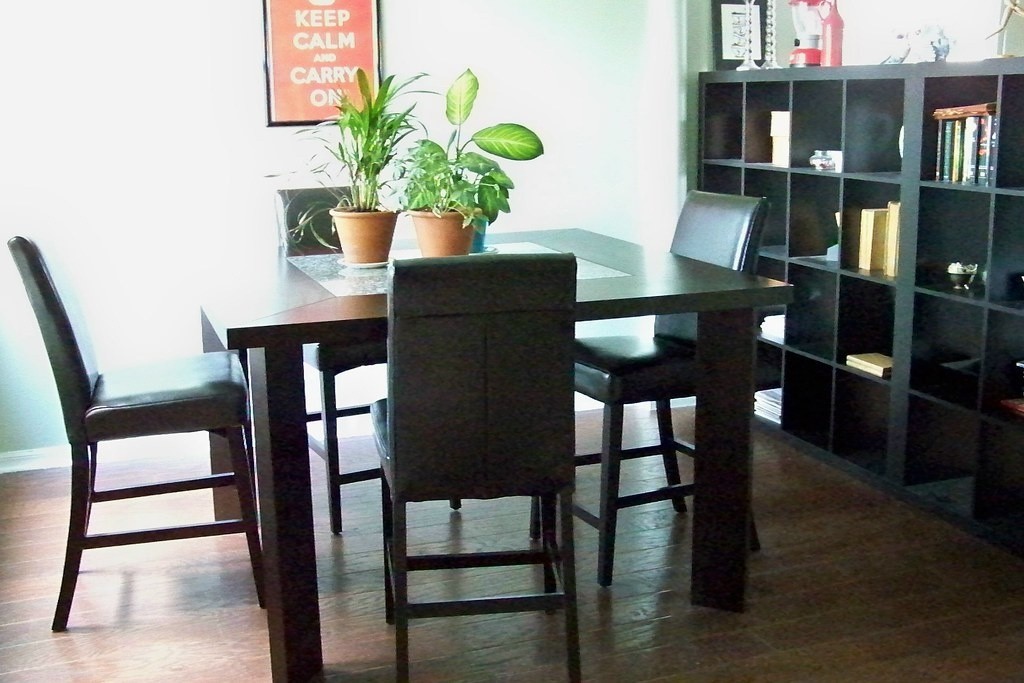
205 229 793 683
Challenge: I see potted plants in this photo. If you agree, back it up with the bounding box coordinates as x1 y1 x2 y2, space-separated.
265 68 544 263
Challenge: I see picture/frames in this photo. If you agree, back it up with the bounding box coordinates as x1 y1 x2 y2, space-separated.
263 0 381 127
711 0 768 70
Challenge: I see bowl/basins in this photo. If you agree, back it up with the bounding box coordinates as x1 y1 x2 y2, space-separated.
948 272 976 290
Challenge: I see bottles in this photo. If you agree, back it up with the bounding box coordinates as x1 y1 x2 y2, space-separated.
818 0 844 66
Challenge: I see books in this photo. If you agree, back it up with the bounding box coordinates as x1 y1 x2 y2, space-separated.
846 352 892 380
759 314 785 345
932 102 997 187
753 387 782 425
858 209 887 271
882 200 900 278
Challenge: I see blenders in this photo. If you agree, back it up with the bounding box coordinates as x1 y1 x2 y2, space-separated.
788 0 825 67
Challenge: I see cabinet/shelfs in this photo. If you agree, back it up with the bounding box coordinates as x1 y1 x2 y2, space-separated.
695 57 1024 560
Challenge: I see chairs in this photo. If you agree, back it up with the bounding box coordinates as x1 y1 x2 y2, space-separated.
370 253 583 683
8 235 266 632
276 188 461 531
538 189 770 585
